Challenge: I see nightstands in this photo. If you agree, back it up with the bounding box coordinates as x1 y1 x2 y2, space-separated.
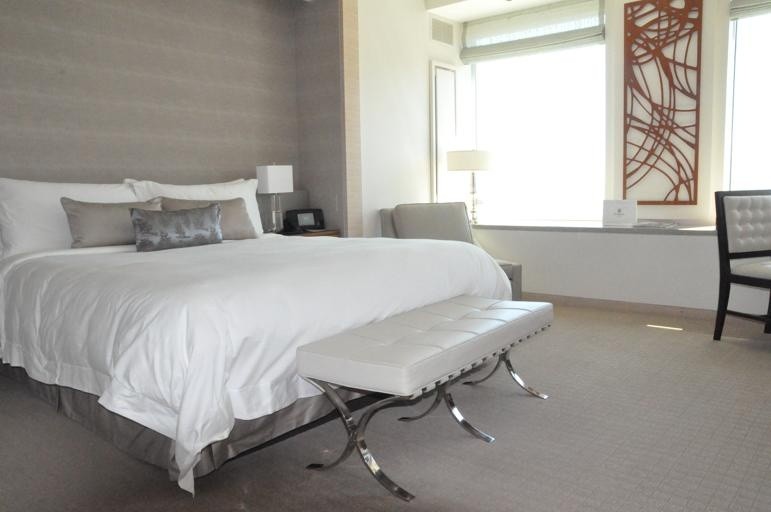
299 228 340 238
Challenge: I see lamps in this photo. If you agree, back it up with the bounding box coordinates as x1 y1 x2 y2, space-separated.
446 149 491 225
256 160 295 234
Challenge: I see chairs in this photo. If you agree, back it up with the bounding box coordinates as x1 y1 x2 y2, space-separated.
375 201 523 301
709 188 770 343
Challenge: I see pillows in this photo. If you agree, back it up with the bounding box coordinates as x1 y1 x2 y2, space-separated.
153 193 260 243
58 195 163 250
123 173 265 235
125 199 225 252
0 176 139 264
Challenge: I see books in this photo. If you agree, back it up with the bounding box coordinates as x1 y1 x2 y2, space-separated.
633 221 677 230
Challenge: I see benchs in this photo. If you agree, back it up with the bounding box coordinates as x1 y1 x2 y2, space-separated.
293 291 559 503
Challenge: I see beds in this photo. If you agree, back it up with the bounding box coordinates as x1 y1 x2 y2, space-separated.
0 233 515 501
